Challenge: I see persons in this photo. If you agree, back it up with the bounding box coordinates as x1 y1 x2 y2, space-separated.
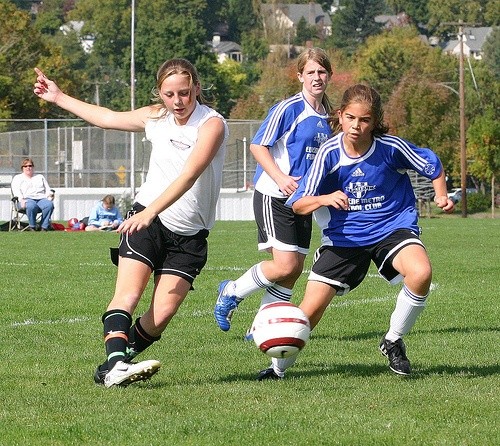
34 58 229 389
84 194 124 231
257 84 455 379
11 159 54 232
215 47 334 345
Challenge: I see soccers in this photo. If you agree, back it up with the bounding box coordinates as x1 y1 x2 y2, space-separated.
252 301 311 358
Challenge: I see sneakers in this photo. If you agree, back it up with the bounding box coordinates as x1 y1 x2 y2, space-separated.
257 368 281 381
379 333 412 375
94 359 160 388
213 280 244 331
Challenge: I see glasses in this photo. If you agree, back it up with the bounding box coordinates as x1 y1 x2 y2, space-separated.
21 164 34 168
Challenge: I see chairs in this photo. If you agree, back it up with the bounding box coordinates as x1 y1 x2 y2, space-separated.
7 188 56 231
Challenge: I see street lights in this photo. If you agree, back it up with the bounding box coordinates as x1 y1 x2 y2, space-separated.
444 19 481 218
86 77 109 106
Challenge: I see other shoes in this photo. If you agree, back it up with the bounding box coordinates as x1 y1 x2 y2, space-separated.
242 330 254 341
105 227 112 231
40 228 46 231
29 227 36 231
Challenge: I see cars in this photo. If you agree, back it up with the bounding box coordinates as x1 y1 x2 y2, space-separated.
446 187 478 205
